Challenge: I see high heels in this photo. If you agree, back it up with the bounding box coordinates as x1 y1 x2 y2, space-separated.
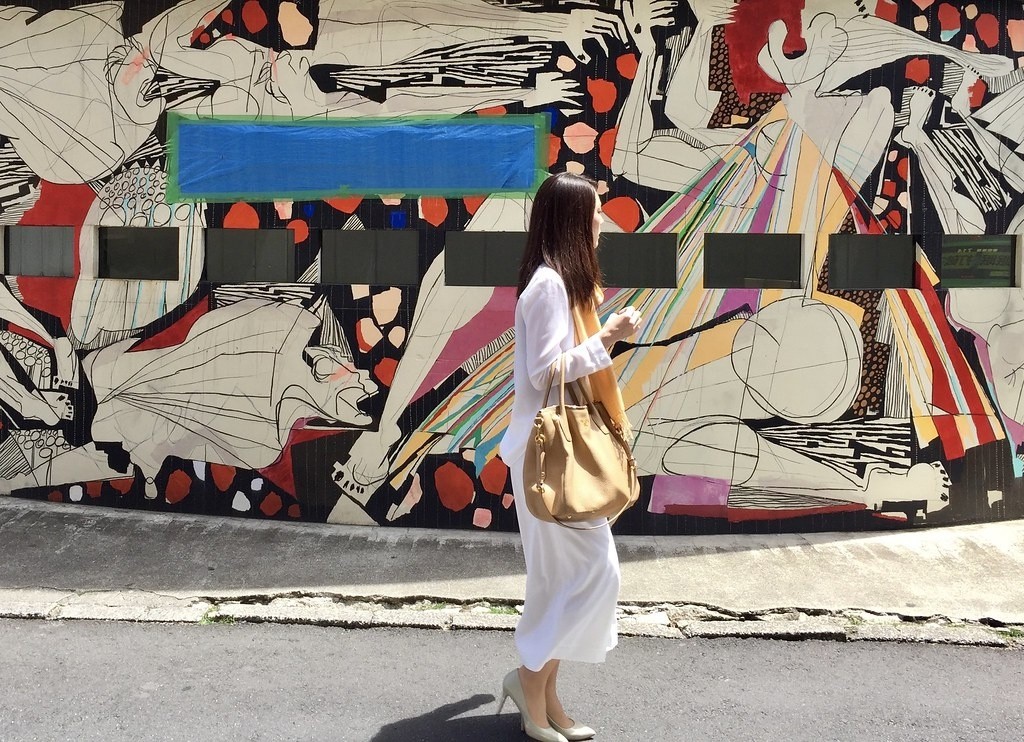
495 668 568 742
519 707 596 740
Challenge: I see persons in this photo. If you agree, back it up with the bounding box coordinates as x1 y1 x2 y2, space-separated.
497 171 643 742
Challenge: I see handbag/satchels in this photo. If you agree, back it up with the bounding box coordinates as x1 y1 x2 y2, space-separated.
523 354 640 530
